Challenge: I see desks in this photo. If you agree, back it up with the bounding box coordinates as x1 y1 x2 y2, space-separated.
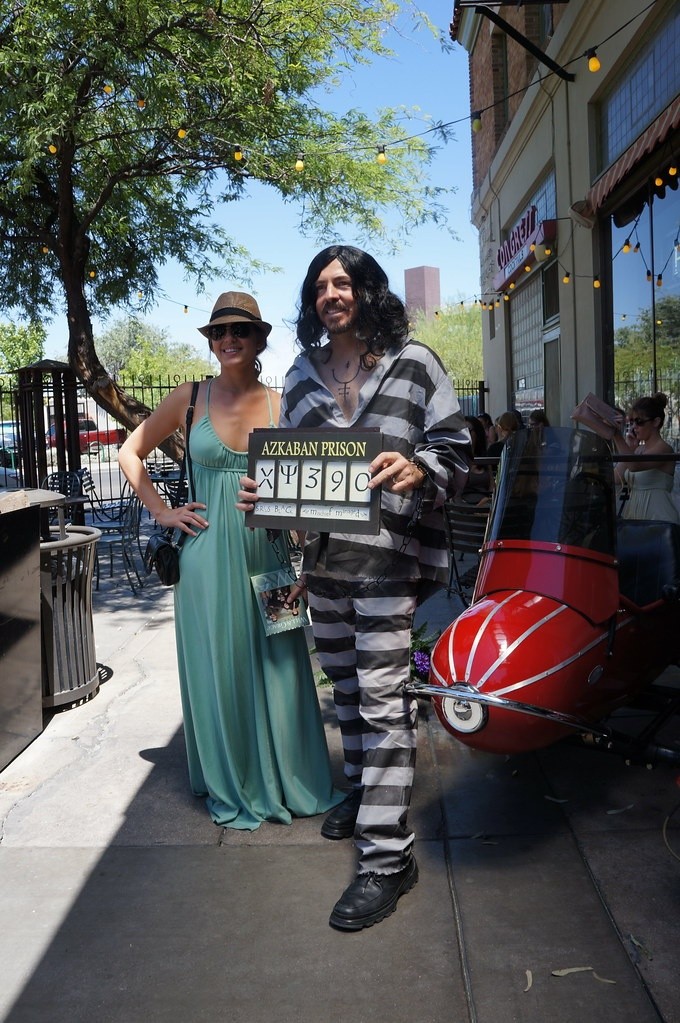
49 497 89 527
148 472 189 511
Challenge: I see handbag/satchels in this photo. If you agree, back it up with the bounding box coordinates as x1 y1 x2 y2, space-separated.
571 392 623 441
143 533 180 586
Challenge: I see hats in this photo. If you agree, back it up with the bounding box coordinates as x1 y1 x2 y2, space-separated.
197 292 272 337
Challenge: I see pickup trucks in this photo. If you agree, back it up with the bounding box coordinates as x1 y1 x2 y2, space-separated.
45 418 127 457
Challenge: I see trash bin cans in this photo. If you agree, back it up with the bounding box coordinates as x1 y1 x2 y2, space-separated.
41 490 102 710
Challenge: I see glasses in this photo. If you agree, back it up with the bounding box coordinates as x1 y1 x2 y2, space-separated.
468 428 473 433
208 323 249 340
478 412 484 417
528 423 536 427
629 417 656 427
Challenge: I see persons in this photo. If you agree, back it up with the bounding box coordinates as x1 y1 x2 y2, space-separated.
119 292 331 827
262 586 300 622
611 392 678 523
236 244 472 930
462 410 564 507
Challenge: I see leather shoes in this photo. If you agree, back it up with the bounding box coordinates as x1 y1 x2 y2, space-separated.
330 856 418 930
322 786 367 837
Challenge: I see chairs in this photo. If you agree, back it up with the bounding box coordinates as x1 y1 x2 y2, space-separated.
40 462 189 596
443 502 488 608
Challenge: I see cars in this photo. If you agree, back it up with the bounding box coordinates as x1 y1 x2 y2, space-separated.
0 462 24 491
0 420 49 470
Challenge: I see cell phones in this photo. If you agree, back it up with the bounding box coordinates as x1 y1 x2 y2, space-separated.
626 428 637 443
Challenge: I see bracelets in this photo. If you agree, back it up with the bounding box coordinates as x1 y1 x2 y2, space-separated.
299 578 309 589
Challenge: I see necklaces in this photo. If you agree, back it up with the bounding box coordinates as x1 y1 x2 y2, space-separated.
332 351 360 369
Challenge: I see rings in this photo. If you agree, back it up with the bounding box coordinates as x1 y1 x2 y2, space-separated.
392 478 399 484
